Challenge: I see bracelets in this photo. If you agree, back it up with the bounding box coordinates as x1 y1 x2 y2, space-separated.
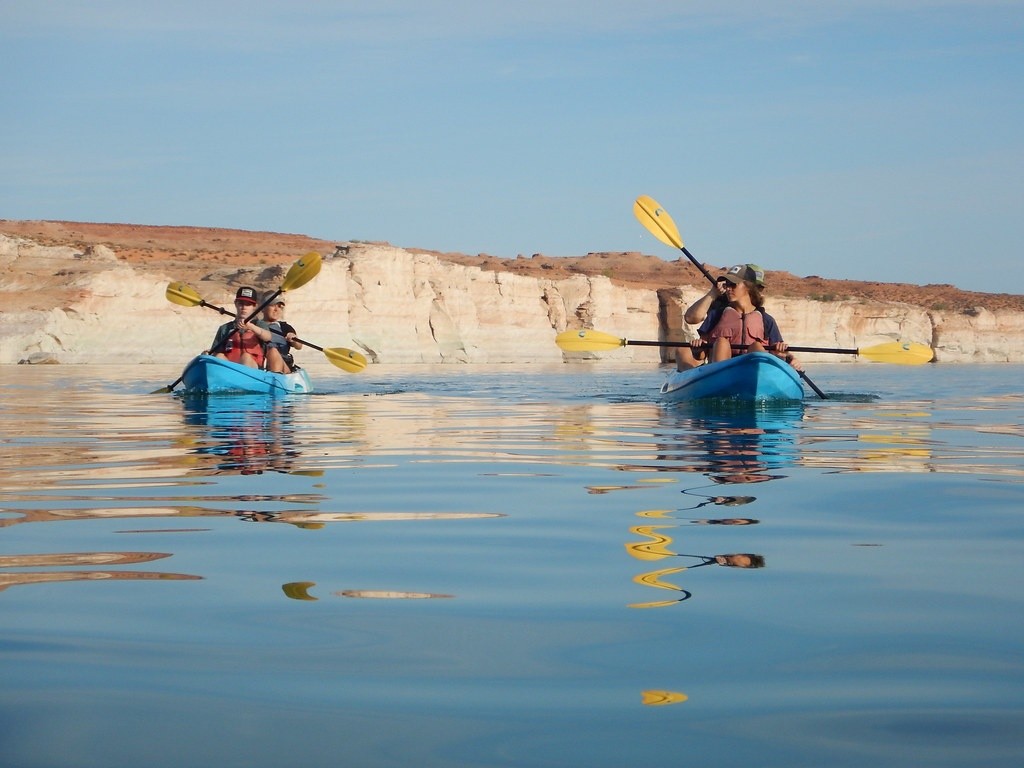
257 328 263 335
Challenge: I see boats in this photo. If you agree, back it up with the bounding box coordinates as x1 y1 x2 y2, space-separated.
658 351 806 414
181 354 315 400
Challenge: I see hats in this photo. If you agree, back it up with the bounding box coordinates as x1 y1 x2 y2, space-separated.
717 264 756 284
746 264 767 288
261 290 286 307
235 287 257 303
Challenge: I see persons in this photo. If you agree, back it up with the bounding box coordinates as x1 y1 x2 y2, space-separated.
258 290 302 374
676 264 803 372
201 286 273 370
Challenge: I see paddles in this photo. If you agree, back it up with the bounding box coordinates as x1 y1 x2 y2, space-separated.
165 282 368 373
148 251 322 394
553 328 935 366
632 194 828 400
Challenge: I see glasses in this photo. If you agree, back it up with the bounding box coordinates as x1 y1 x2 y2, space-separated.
722 282 743 289
237 300 256 307
